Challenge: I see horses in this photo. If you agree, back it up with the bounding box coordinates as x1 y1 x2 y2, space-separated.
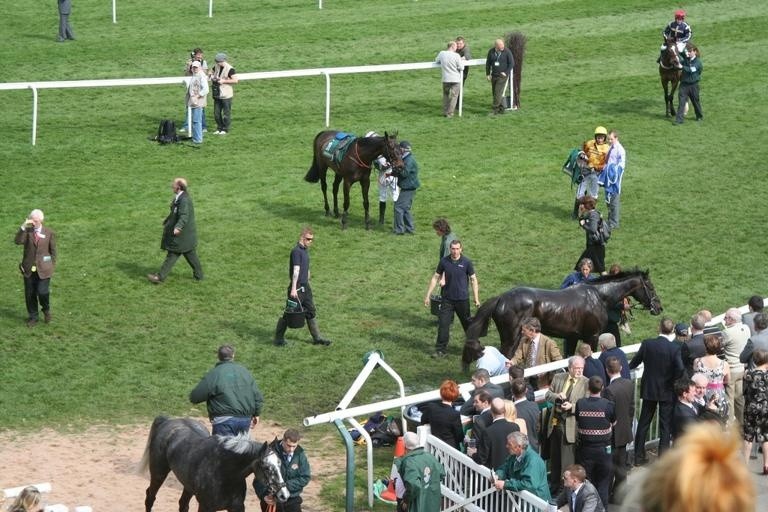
303 129 404 231
659 35 682 118
467 265 663 360
134 415 291 512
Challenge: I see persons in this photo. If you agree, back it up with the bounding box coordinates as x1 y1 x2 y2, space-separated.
209 53 238 135
384 140 421 236
420 380 464 451
505 318 562 369
721 307 751 420
597 333 631 386
57 0 75 41
364 131 399 224
672 378 718 441
179 47 209 132
462 339 508 377
424 240 481 358
573 126 610 217
395 432 446 512
273 227 332 346
486 39 515 115
675 323 689 344
662 10 692 43
693 335 733 417
189 345 264 438
672 45 704 125
464 389 492 448
4 486 44 512
739 314 768 370
432 219 459 287
697 310 725 360
610 264 631 335
602 356 635 495
504 398 527 435
467 397 520 471
558 257 595 357
147 177 203 285
511 380 541 454
575 375 617 509
681 315 706 378
505 365 535 402
578 343 606 387
603 129 626 229
692 373 719 413
575 196 606 276
629 318 684 466
185 61 209 144
252 428 312 512
488 431 551 505
742 295 764 332
619 422 758 511
549 464 606 512
742 348 768 474
14 209 56 327
455 36 470 110
435 41 464 118
460 368 504 415
545 355 589 494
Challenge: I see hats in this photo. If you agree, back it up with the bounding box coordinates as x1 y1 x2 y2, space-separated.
404 432 420 449
215 53 227 62
192 61 200 68
674 324 689 335
400 140 412 152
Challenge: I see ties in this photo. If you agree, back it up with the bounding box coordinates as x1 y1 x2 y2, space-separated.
525 340 533 368
566 379 574 402
286 453 291 465
698 399 704 405
35 231 38 242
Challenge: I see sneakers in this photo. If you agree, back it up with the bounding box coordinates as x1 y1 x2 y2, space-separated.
672 121 683 126
213 131 219 135
179 127 187 132
45 313 51 323
698 117 703 121
27 318 38 327
145 274 161 283
431 352 448 359
764 466 768 475
203 130 207 132
219 131 226 135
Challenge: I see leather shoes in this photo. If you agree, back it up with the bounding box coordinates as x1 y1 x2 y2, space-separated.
635 455 649 467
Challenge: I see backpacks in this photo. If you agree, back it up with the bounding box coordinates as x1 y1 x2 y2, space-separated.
598 218 611 246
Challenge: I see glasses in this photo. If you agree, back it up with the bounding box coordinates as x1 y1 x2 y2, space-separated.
563 474 569 480
304 237 313 240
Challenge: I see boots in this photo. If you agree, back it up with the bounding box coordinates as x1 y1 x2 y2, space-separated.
273 317 287 347
379 201 386 224
574 198 581 219
306 316 332 346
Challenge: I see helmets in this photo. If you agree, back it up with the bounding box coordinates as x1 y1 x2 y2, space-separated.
594 126 607 136
675 10 685 19
